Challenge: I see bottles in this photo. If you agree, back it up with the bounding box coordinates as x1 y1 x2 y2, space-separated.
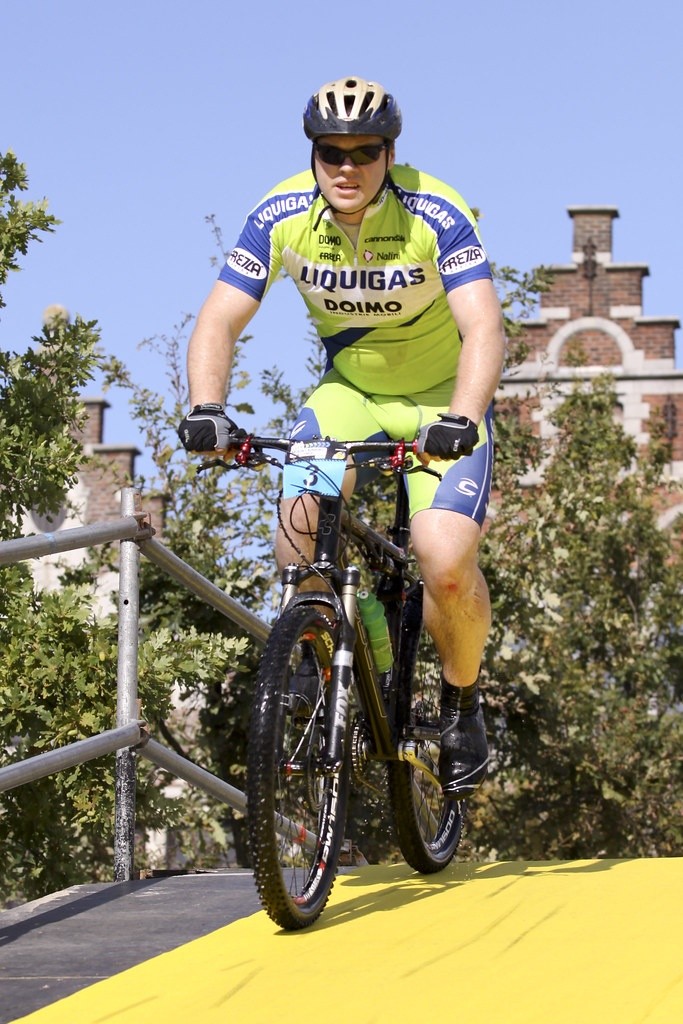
358 591 394 674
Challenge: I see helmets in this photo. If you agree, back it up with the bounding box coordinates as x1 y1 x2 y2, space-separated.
303 77 403 141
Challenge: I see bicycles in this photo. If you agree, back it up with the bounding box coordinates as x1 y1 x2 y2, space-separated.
194 431 470 930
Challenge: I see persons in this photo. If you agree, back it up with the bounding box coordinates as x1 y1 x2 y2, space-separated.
178 75 507 799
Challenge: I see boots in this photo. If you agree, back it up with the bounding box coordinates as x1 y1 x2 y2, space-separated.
289 638 324 716
439 670 491 798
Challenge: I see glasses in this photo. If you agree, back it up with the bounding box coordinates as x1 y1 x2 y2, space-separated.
311 142 388 166
